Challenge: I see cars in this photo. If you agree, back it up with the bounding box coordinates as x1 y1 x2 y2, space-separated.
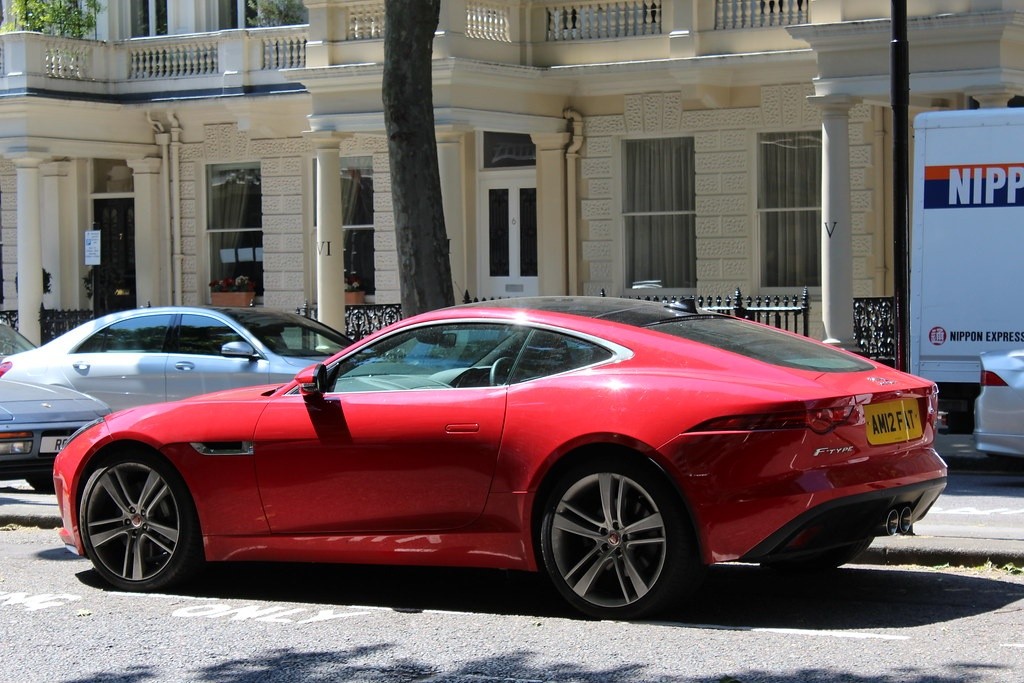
0 312 362 493
974 348 1023 453
54 297 949 620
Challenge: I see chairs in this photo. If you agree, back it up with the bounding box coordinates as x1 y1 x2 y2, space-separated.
509 339 611 386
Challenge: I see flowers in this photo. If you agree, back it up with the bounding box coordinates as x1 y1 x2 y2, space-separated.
209 276 255 292
343 274 364 291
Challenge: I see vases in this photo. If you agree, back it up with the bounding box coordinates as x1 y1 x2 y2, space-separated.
345 292 364 304
211 293 255 307
